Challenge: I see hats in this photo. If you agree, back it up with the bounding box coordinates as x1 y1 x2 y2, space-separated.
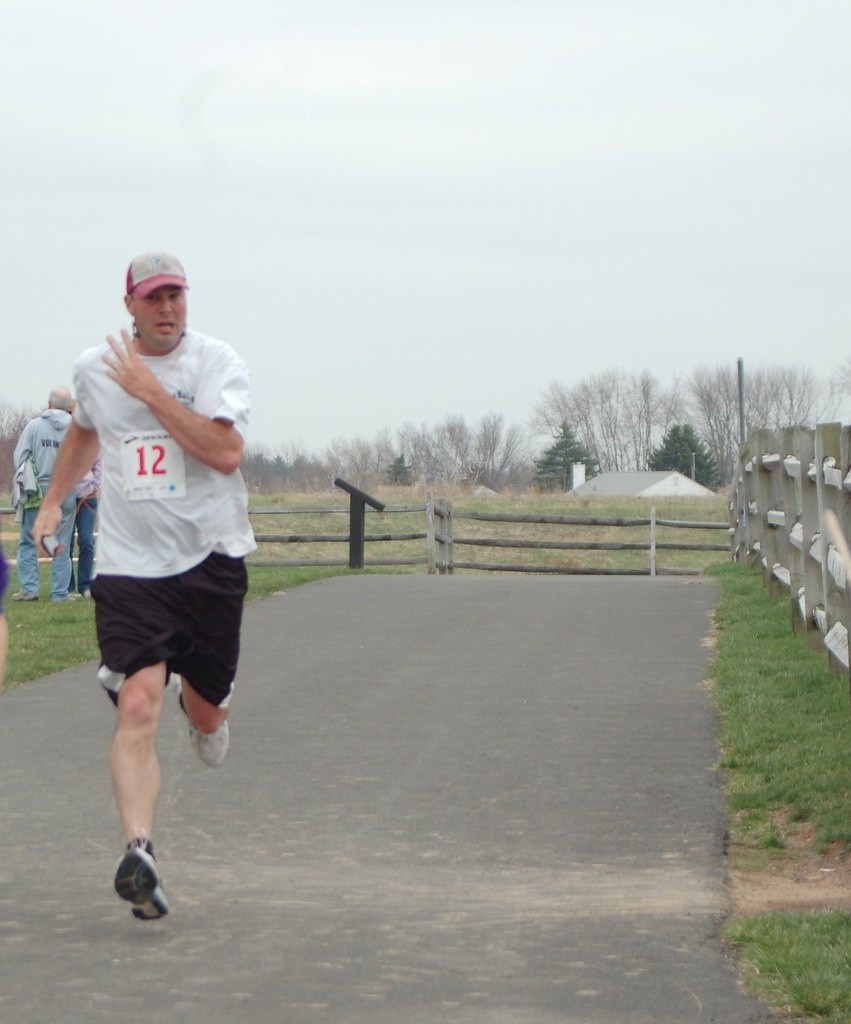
127 245 189 300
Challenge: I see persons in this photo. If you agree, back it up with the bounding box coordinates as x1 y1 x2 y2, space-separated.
11 386 78 602
63 398 103 598
33 251 254 926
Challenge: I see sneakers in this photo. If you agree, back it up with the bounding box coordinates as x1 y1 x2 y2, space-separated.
169 670 231 766
113 847 169 919
13 591 38 601
